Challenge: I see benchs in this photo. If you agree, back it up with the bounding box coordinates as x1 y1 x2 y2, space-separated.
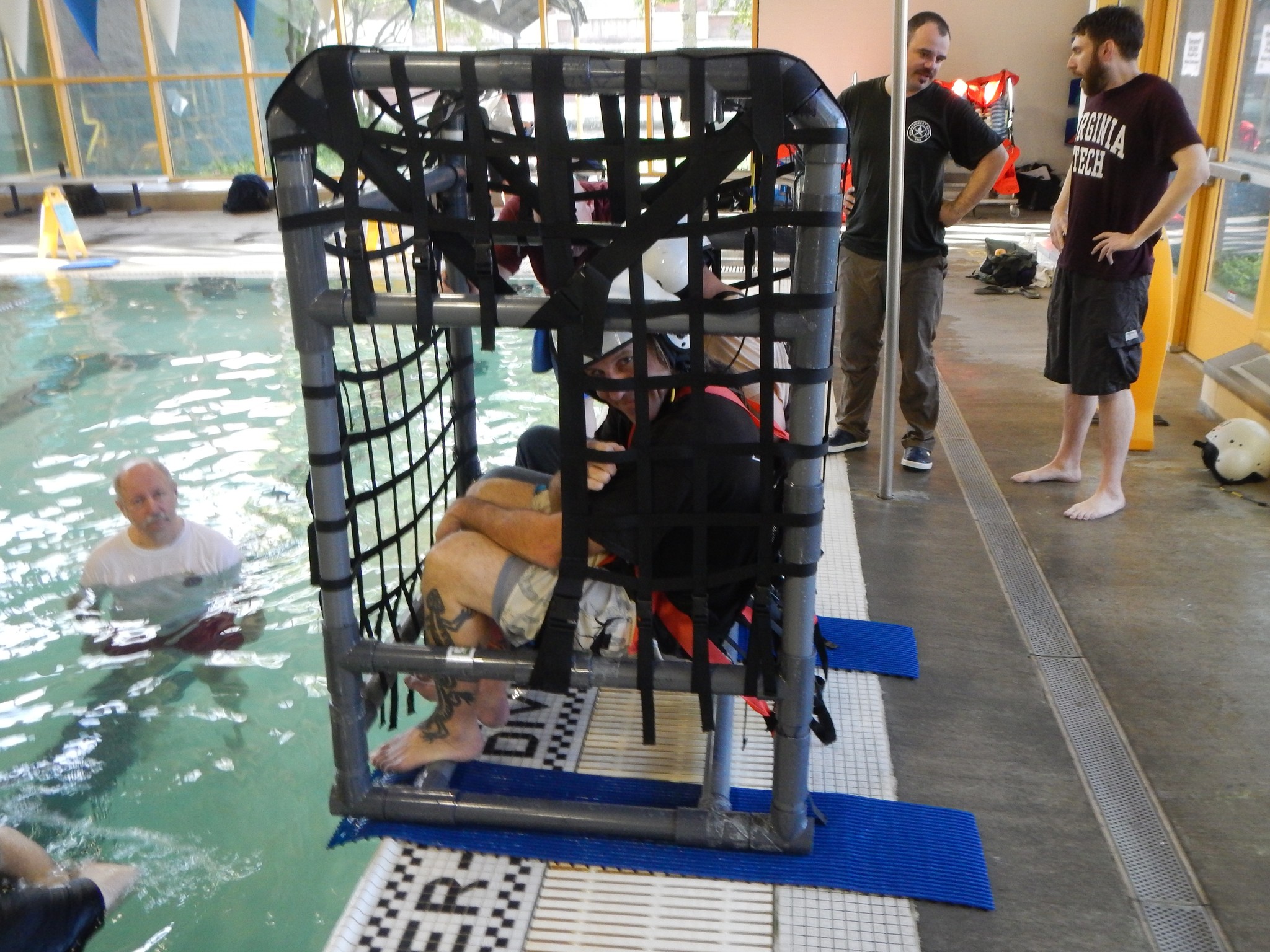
1198 341 1270 428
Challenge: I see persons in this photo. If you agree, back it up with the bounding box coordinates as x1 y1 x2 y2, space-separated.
1009 5 1212 521
65 458 267 654
440 178 611 296
0 818 141 952
824 10 1009 471
479 210 791 490
365 268 788 772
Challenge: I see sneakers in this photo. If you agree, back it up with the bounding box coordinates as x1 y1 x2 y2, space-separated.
900 442 934 473
824 427 868 454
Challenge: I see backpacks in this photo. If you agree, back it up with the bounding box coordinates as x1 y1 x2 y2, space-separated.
973 237 1034 286
69 180 107 217
222 173 270 215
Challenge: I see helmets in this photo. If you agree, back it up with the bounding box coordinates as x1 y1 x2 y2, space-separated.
541 259 696 373
1206 414 1269 487
616 198 715 295
